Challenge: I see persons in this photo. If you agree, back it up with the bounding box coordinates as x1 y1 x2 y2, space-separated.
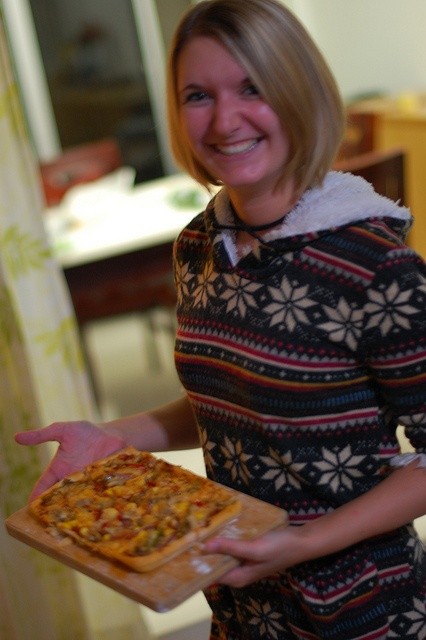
13 0 425 640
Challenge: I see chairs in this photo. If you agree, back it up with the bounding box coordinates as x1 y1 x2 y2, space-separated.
38 133 122 205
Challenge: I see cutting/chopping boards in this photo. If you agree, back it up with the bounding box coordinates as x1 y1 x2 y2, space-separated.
4 449 288 613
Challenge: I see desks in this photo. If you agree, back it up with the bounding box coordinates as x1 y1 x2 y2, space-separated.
62 170 220 418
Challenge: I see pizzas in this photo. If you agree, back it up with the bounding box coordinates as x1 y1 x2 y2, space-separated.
29 448 240 573
